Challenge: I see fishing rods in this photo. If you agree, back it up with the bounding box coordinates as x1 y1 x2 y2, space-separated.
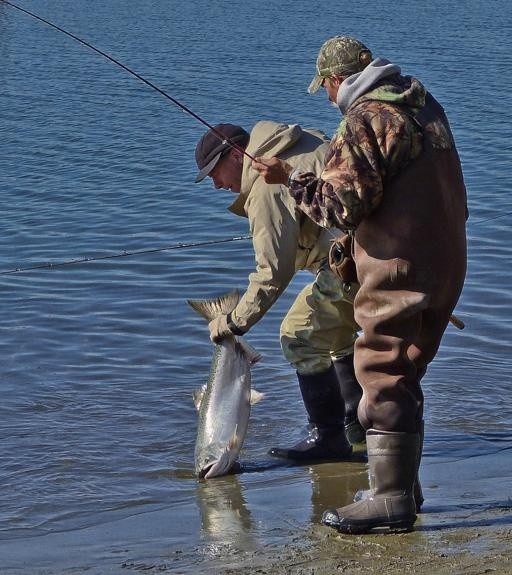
2 0 464 330
1 235 251 274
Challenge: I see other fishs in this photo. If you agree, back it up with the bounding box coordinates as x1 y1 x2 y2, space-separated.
186 286 266 481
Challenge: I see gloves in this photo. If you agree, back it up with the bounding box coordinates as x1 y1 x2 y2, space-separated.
208 315 232 344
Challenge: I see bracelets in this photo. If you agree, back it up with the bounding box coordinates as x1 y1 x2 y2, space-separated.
225 312 244 338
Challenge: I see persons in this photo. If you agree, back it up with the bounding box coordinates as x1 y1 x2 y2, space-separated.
193 116 366 462
245 30 471 536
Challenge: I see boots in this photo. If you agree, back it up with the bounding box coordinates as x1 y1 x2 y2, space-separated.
268 352 360 464
322 422 424 535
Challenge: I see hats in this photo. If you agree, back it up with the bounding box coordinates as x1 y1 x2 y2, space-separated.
307 36 371 93
195 123 249 183
